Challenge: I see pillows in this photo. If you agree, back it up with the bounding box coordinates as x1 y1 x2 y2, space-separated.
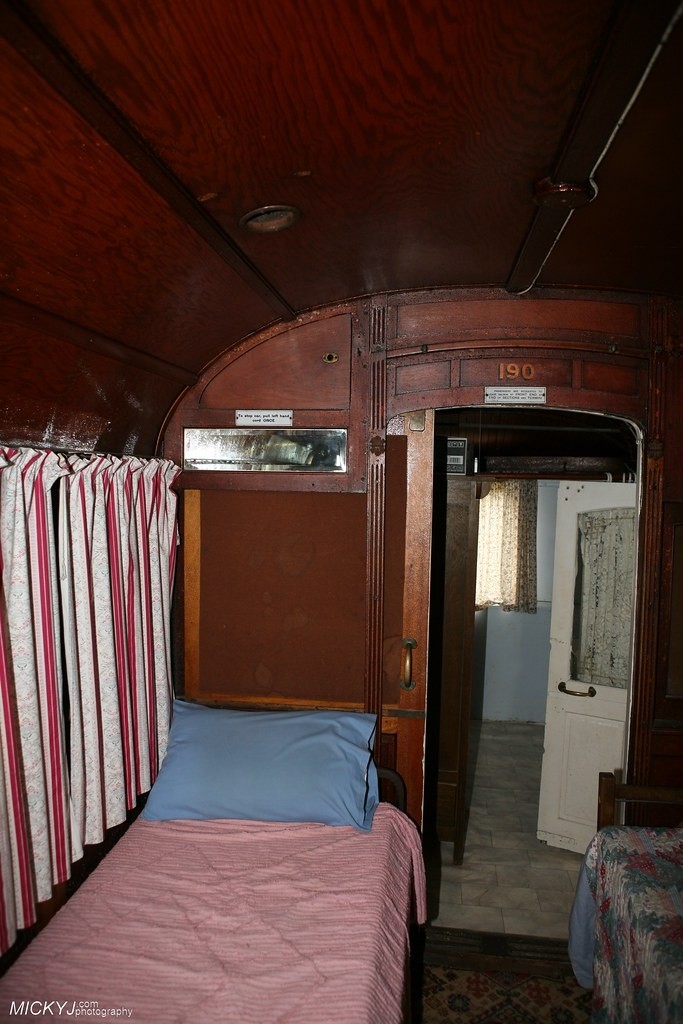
144 697 382 835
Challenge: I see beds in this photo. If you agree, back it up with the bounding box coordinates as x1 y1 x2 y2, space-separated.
567 772 683 1024
0 769 425 1023
